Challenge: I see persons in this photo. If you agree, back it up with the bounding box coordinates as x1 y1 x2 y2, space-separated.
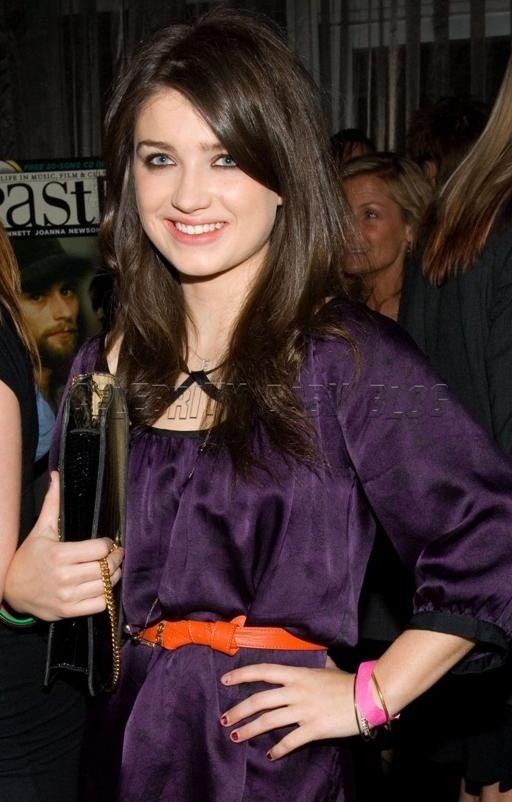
0 17 511 802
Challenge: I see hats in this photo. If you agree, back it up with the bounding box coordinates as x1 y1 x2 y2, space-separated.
10 237 82 289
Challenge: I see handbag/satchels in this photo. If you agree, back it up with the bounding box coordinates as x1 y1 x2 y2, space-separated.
40 371 133 704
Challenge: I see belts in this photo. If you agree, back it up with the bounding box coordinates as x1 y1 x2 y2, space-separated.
141 616 327 655
